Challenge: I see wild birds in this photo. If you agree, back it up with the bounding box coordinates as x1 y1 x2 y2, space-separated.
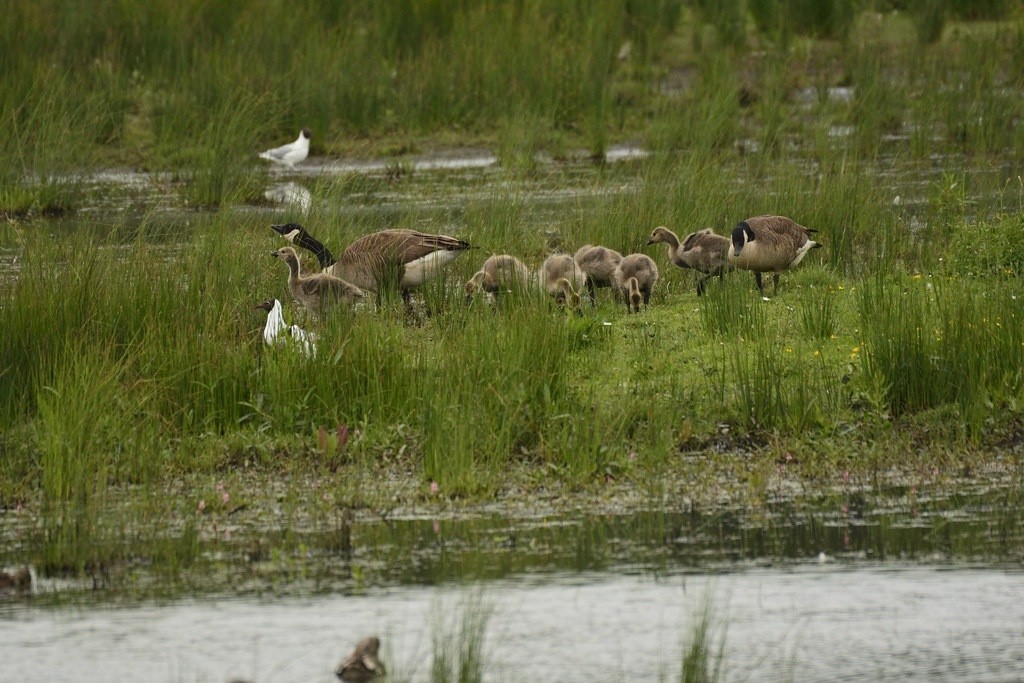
255 299 318 362
573 242 658 313
464 254 528 303
727 215 823 293
257 128 311 170
537 255 586 317
646 226 734 297
269 246 362 314
271 223 479 305
